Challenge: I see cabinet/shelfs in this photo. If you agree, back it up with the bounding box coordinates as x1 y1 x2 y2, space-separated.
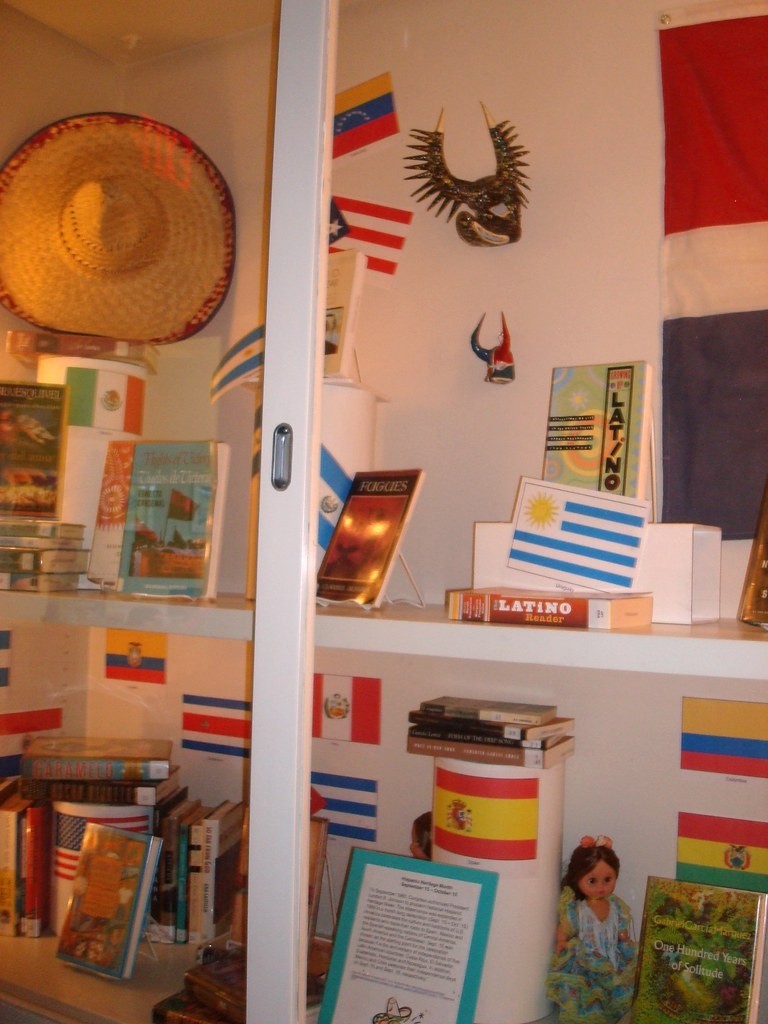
0 1 768 1024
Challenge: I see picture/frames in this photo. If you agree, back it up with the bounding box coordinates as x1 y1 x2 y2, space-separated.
314 847 499 1024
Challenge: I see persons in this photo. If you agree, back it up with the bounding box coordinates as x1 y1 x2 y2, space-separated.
545 834 640 1024
408 810 432 861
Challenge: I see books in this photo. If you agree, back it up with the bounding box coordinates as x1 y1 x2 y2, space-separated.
0 733 334 1024
323 247 369 381
632 875 768 1024
315 467 425 607
443 589 657 630
407 695 577 772
317 846 500 1023
0 332 231 601
542 361 656 504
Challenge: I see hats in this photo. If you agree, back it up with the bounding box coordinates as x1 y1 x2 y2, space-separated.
0 111 237 346
